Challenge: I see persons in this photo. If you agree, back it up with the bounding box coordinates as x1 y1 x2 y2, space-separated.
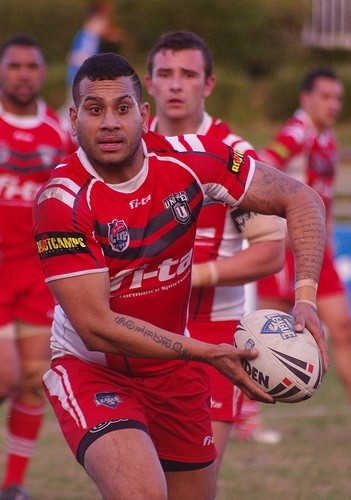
142 31 288 499
0 36 88 498
65 1 131 101
33 54 328 500
232 68 351 445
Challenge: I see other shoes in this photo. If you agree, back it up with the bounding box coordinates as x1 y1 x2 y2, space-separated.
0 486 33 500
229 419 281 444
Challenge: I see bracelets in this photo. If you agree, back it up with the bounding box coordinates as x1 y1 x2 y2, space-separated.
292 278 318 311
190 261 219 288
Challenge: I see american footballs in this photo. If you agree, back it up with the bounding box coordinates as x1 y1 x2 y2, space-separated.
234 311 322 403
